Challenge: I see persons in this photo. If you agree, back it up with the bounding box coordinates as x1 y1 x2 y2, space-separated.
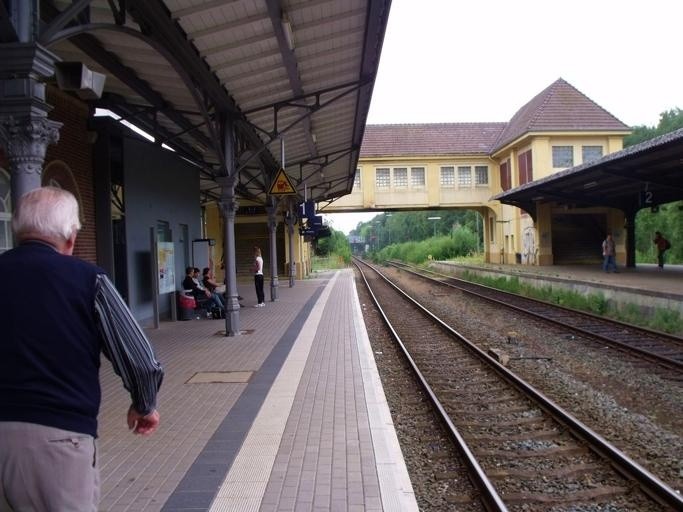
192 267 226 313
653 230 668 269
0 183 165 511
182 267 227 320
201 268 245 309
601 233 622 274
251 246 266 308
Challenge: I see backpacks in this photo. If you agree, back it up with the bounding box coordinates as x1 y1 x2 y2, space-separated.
213 306 225 319
665 240 670 250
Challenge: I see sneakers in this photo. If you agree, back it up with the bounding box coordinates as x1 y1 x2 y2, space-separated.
206 312 212 319
239 295 266 308
